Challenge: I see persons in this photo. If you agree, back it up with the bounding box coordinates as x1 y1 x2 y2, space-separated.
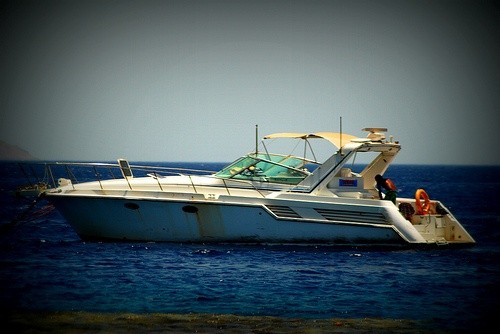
374 173 399 207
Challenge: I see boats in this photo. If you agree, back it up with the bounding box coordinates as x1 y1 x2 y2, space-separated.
21 115 477 245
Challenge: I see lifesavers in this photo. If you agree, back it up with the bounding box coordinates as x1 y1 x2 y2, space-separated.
414 189 431 214
